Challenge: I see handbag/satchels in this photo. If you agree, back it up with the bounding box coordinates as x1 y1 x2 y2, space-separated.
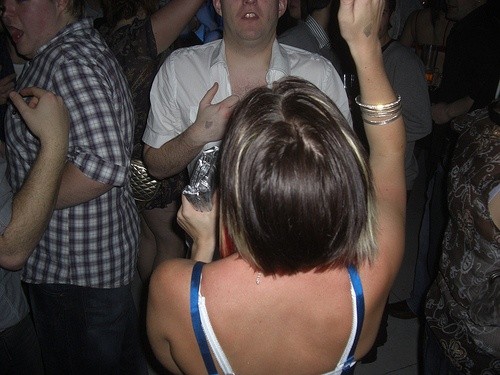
183 146 220 247
129 159 161 202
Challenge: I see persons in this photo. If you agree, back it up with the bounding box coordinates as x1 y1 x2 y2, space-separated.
85 1 483 319
415 98 500 374
0 87 73 375
143 0 352 180
148 0 407 375
1 0 142 375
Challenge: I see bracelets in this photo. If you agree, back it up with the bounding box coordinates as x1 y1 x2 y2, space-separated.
355 94 403 127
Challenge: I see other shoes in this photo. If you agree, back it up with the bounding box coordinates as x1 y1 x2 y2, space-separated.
388 300 417 319
